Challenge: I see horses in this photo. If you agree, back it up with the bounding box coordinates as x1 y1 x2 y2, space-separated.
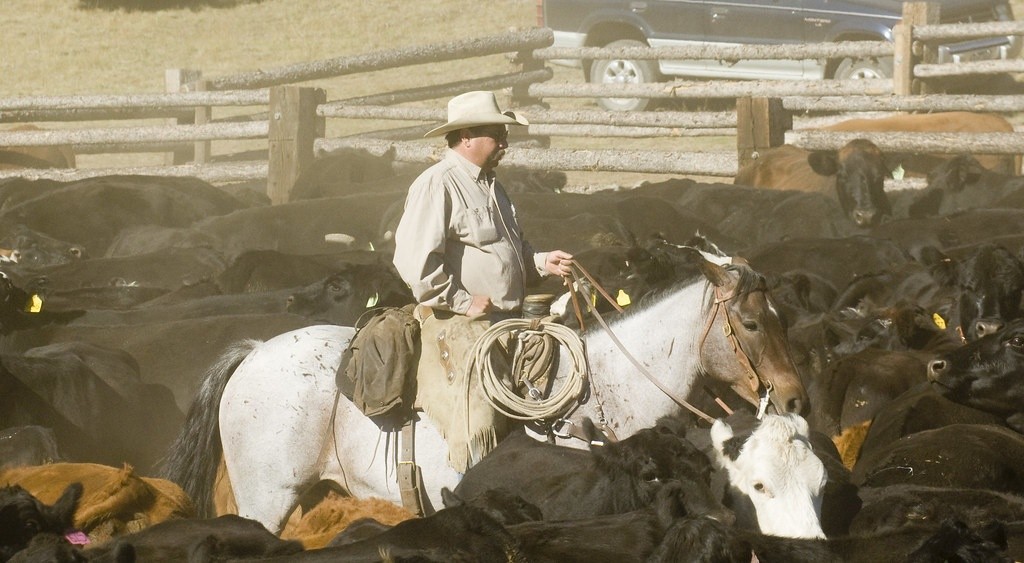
2 111 1024 563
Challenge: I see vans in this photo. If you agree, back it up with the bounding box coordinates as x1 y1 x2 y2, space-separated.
544 0 1023 114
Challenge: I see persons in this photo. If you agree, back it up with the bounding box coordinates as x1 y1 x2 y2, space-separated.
390 90 574 472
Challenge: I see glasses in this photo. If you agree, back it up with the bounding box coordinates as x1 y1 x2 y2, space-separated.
477 129 508 141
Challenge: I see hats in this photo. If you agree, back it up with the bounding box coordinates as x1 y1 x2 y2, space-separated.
423 92 529 139
